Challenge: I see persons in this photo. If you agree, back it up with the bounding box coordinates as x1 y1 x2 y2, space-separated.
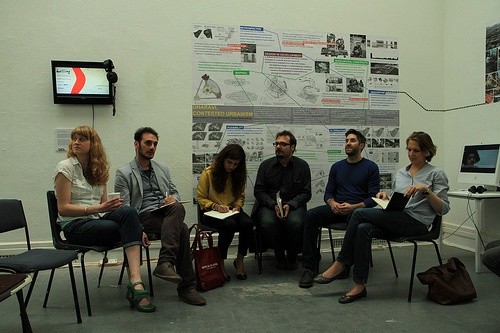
313 132 451 304
53 125 157 312
299 129 381 287
115 127 207 305
462 150 480 168
254 130 312 270
196 144 253 282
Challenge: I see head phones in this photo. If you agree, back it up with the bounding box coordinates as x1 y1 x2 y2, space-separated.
468 185 486 193
103 59 118 83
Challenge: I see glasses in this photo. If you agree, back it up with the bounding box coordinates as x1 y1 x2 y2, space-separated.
272 141 290 146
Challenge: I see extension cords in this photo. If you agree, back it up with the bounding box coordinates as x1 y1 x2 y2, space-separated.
99 258 118 266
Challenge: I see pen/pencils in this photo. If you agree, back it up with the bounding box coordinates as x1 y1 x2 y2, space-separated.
166 191 168 197
220 200 229 212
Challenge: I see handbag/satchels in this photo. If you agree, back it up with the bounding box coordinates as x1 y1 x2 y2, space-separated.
416 256 479 306
193 231 228 292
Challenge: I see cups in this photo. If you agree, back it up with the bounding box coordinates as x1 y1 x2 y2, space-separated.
107 192 120 203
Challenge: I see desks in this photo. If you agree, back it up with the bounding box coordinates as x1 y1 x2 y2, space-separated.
438 190 500 272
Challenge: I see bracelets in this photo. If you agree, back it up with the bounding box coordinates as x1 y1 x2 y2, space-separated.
85 206 87 215
424 189 430 195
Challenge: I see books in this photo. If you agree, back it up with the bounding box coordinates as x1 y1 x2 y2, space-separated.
204 209 240 219
372 192 410 214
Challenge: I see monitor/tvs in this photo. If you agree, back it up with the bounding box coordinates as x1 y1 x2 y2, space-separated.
455 143 500 187
51 60 113 104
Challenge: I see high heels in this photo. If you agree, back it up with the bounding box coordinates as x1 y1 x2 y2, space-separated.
127 278 150 296
125 293 157 313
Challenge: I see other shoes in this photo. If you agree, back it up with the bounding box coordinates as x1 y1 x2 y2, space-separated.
274 251 285 263
152 261 182 284
220 264 231 282
313 269 350 284
177 286 206 307
233 259 247 280
286 256 299 270
298 267 316 287
338 287 368 303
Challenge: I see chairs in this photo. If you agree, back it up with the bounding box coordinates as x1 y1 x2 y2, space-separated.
373 214 442 303
42 190 160 317
316 220 373 269
196 202 265 275
1 268 33 333
0 199 83 323
